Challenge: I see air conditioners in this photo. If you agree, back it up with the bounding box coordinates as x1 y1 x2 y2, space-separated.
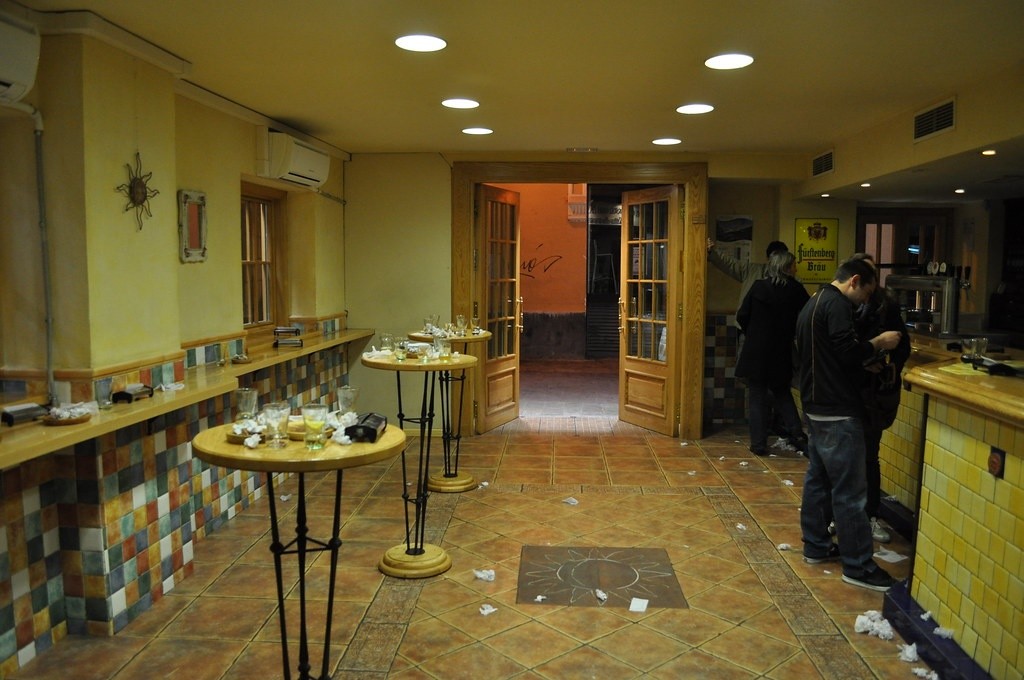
0 4 43 108
266 130 332 188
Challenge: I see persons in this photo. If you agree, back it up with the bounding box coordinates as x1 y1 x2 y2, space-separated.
734 251 811 459
790 258 902 592
826 252 912 547
705 233 792 451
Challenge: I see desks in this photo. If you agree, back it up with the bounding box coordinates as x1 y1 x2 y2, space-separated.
191 416 408 680
407 328 492 493
0 327 376 470
360 350 478 580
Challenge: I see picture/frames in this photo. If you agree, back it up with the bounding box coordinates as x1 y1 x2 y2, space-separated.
177 189 209 265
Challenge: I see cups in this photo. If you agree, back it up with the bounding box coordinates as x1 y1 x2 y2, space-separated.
456 315 468 336
961 339 976 358
231 388 259 423
433 334 447 352
440 341 451 363
444 323 455 336
302 404 328 449
336 387 360 417
424 319 434 335
418 346 432 363
471 318 480 335
971 338 989 359
430 315 440 328
381 333 394 351
395 337 408 361
263 403 291 448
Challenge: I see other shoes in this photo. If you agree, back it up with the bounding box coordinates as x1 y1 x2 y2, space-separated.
750 447 764 455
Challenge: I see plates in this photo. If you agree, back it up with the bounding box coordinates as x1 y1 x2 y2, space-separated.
285 422 335 440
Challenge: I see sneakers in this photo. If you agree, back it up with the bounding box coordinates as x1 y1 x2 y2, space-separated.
841 559 898 591
806 544 840 563
869 517 890 542
